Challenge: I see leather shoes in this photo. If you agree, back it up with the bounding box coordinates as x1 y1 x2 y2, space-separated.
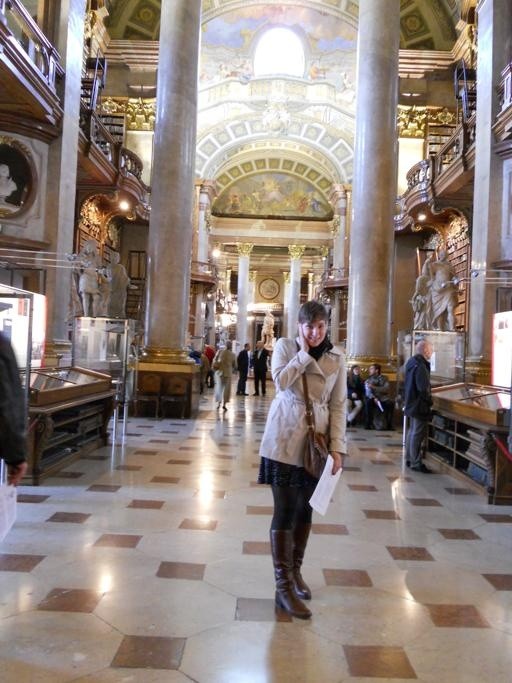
406 460 434 474
236 391 265 396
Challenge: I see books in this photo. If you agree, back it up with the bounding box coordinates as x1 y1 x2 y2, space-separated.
463 428 486 465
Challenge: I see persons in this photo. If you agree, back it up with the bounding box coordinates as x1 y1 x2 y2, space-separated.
190 340 269 409
403 340 434 474
346 365 364 427
0 331 27 486
411 250 464 332
71 239 139 319
397 107 454 136
364 364 394 430
261 310 274 350
100 97 155 131
257 300 347 619
0 163 21 212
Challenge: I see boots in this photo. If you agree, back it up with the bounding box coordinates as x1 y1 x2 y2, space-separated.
270 522 313 619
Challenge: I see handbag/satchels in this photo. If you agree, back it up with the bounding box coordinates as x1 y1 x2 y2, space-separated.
211 362 219 371
305 429 330 480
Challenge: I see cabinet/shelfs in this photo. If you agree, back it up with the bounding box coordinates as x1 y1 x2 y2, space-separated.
16 366 118 486
423 382 512 505
